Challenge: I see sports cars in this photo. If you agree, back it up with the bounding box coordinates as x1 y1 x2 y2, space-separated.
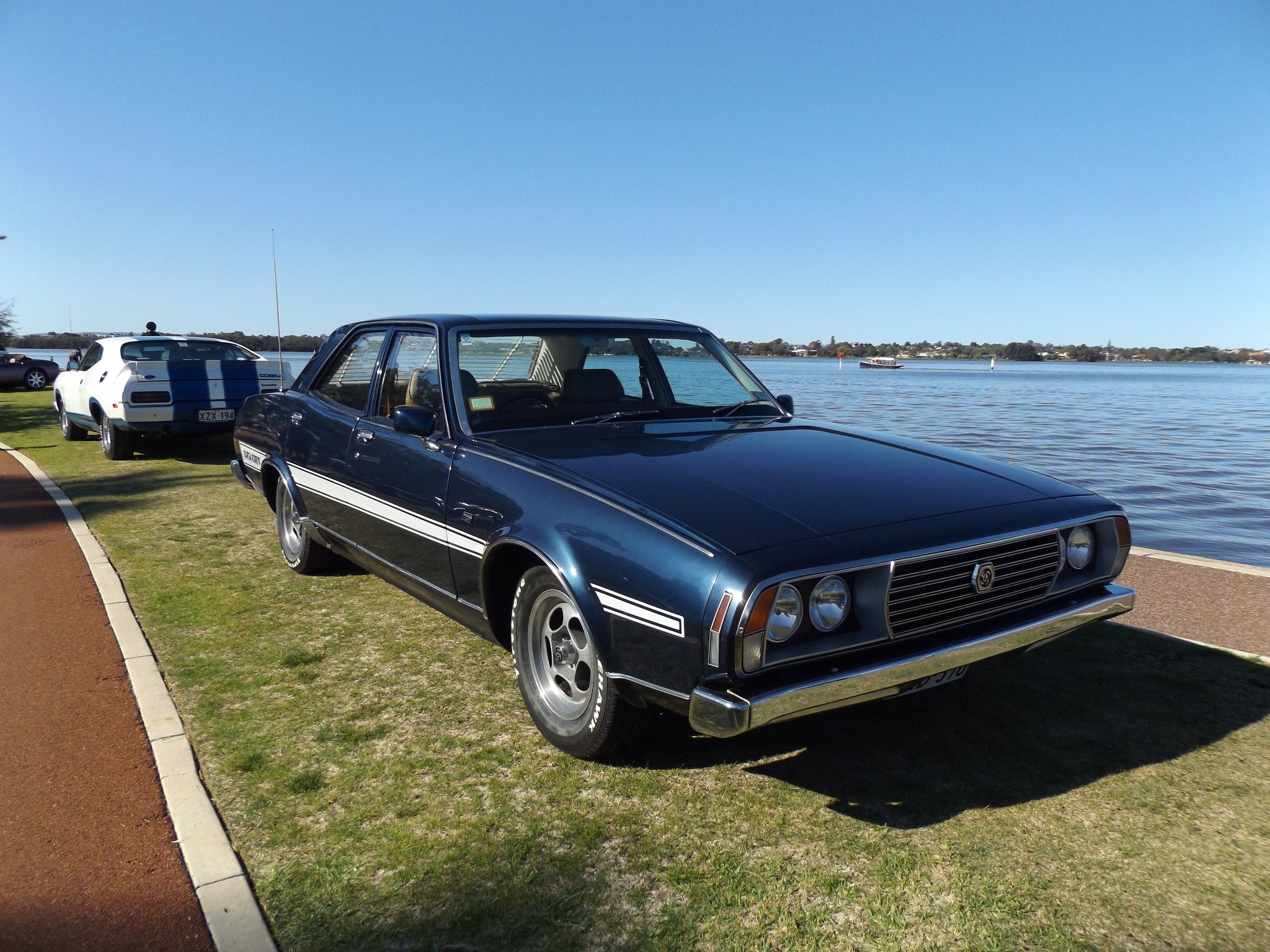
0 354 60 392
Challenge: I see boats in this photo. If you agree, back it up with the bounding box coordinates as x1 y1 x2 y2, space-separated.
858 352 905 370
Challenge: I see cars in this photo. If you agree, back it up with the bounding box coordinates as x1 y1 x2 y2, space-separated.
0 345 9 354
53 336 300 461
231 312 1136 761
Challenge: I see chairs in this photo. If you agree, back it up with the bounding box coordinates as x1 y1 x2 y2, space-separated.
557 367 639 414
406 362 484 418
9 358 16 362
168 345 198 360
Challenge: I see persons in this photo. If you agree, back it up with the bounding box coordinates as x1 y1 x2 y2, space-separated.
69 347 82 362
